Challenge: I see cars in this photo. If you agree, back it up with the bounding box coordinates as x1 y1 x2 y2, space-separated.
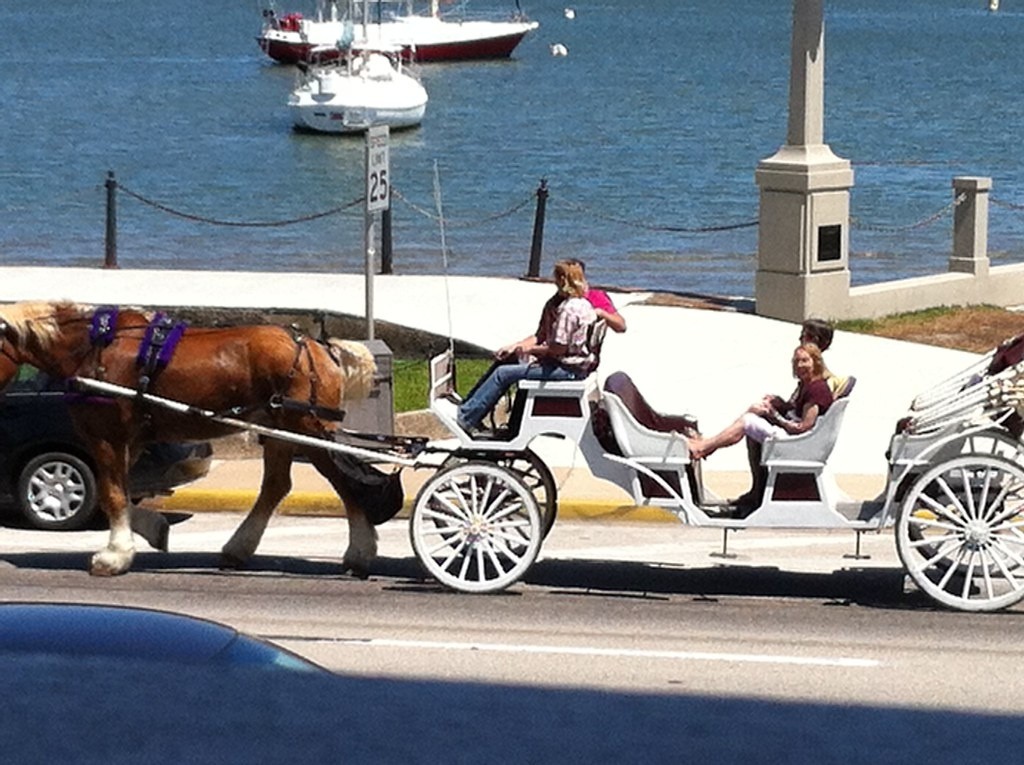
1 602 329 673
0 380 214 531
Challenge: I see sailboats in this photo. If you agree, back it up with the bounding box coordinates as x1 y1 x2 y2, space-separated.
254 0 539 64
285 1 429 135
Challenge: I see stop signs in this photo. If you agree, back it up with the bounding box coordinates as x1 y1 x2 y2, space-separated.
367 125 389 213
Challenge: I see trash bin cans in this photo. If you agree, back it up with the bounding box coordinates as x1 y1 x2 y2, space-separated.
336 339 396 449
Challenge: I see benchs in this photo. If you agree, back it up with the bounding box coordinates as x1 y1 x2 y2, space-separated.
519 317 608 389
762 376 856 461
604 371 698 460
887 374 976 462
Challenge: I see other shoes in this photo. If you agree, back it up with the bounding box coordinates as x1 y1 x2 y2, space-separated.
437 390 474 432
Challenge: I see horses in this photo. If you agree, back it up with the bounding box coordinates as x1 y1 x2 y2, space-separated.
0 301 379 578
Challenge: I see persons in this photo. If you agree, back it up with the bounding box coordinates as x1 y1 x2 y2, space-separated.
680 318 840 507
435 258 626 432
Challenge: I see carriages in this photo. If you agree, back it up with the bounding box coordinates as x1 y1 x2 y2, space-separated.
0 301 1024 614
